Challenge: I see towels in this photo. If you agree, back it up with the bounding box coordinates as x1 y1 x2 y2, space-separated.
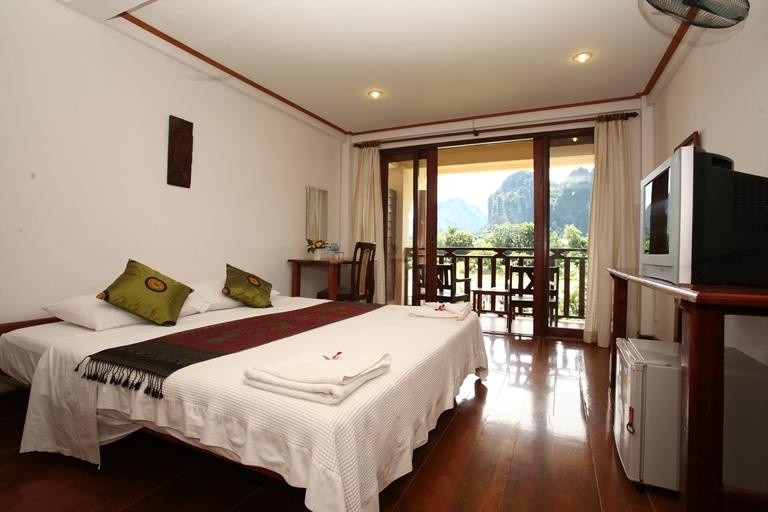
243 341 393 405
410 303 473 321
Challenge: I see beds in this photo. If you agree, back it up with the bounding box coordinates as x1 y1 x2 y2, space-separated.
1 294 488 512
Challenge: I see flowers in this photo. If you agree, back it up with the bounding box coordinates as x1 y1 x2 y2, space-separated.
305 239 328 253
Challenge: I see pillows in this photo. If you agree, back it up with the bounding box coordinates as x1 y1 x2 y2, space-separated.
42 281 205 331
96 259 194 326
195 284 281 314
221 263 273 307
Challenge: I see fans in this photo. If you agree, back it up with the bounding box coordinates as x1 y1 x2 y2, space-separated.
646 1 752 27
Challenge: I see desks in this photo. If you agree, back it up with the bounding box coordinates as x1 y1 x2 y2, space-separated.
472 289 515 320
286 257 377 301
606 268 768 512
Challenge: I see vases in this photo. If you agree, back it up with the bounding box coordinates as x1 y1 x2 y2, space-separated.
313 249 321 261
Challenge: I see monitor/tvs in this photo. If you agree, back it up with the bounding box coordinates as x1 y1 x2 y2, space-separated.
639 146 768 289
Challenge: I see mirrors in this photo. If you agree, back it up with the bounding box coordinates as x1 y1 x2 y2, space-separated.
305 185 328 241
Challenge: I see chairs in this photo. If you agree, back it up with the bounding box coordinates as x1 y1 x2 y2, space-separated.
414 265 471 303
507 267 560 332
318 242 375 303
